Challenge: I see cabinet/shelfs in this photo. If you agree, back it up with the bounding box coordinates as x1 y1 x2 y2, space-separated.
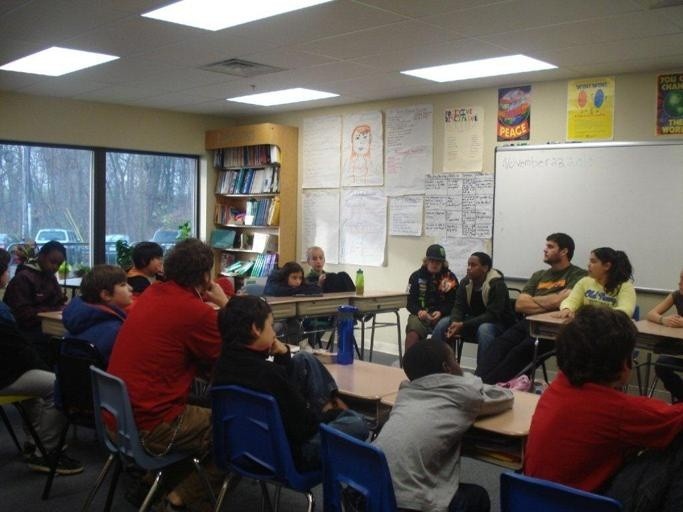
206 121 300 283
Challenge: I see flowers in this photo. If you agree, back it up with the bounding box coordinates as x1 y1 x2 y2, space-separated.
11 240 38 264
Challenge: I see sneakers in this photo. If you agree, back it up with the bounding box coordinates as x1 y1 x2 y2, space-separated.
23 443 85 474
124 478 150 508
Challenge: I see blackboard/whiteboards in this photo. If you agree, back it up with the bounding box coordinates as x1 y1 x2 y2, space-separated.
493 141 683 294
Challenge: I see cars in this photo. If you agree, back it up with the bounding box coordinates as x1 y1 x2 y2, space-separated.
149 229 180 251
0 233 19 264
104 235 129 259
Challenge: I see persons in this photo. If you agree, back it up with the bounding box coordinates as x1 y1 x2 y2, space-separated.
551 244 638 325
648 270 683 405
347 337 513 511
476 232 590 382
0 232 375 512
523 302 683 511
403 241 462 377
431 247 514 377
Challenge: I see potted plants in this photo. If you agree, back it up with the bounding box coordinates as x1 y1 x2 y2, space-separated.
58 262 71 279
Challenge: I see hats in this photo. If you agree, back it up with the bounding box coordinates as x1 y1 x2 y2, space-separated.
426 245 445 261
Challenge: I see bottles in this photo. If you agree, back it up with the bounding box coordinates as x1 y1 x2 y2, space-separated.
355 269 364 296
336 305 358 365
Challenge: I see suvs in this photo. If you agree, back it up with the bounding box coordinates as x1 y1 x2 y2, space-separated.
34 228 82 262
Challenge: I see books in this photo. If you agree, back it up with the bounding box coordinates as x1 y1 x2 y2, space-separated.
213 144 281 279
